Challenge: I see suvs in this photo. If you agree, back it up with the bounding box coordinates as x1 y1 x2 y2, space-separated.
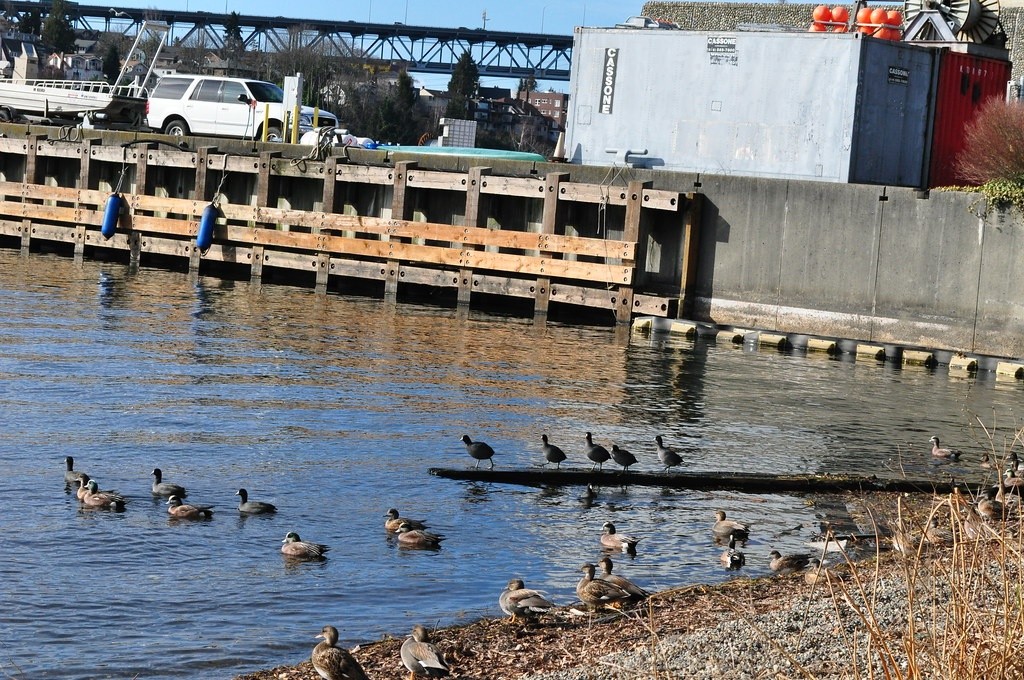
146 74 338 158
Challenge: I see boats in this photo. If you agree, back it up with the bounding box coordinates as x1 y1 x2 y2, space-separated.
0 21 174 119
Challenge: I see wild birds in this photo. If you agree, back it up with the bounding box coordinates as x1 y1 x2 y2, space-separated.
541 434 567 470
767 550 847 584
164 494 215 519
893 435 1024 555
499 579 556 622
611 445 639 472
281 532 331 556
712 511 750 568
585 432 612 472
75 473 125 508
382 508 447 547
311 626 371 680
234 488 278 513
150 469 187 498
64 456 90 482
460 435 495 469
652 436 685 472
581 483 639 549
400 626 451 680
576 558 648 613
